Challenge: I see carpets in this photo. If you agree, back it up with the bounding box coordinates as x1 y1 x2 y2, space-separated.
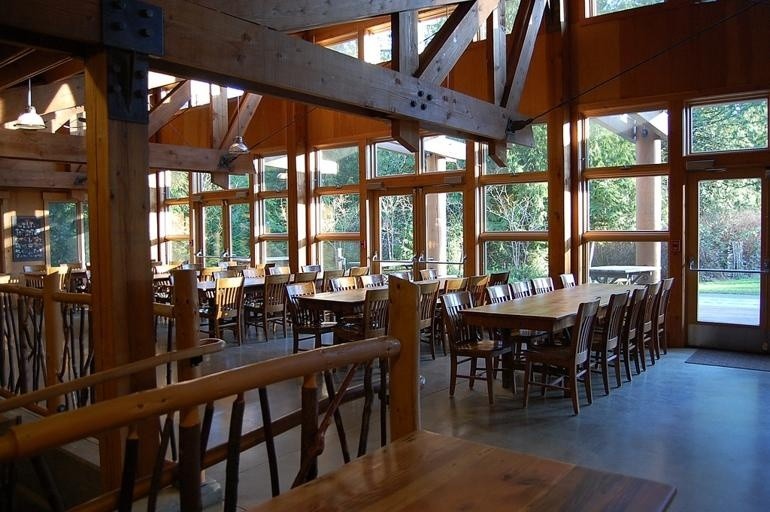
685 348 770 371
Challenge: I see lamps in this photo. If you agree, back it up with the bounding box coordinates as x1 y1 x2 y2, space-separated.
14 78 46 130
228 90 248 155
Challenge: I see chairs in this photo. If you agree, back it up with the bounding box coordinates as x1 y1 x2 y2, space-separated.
8 263 675 416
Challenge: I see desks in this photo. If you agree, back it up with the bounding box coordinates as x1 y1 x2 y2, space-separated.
235 428 676 512
